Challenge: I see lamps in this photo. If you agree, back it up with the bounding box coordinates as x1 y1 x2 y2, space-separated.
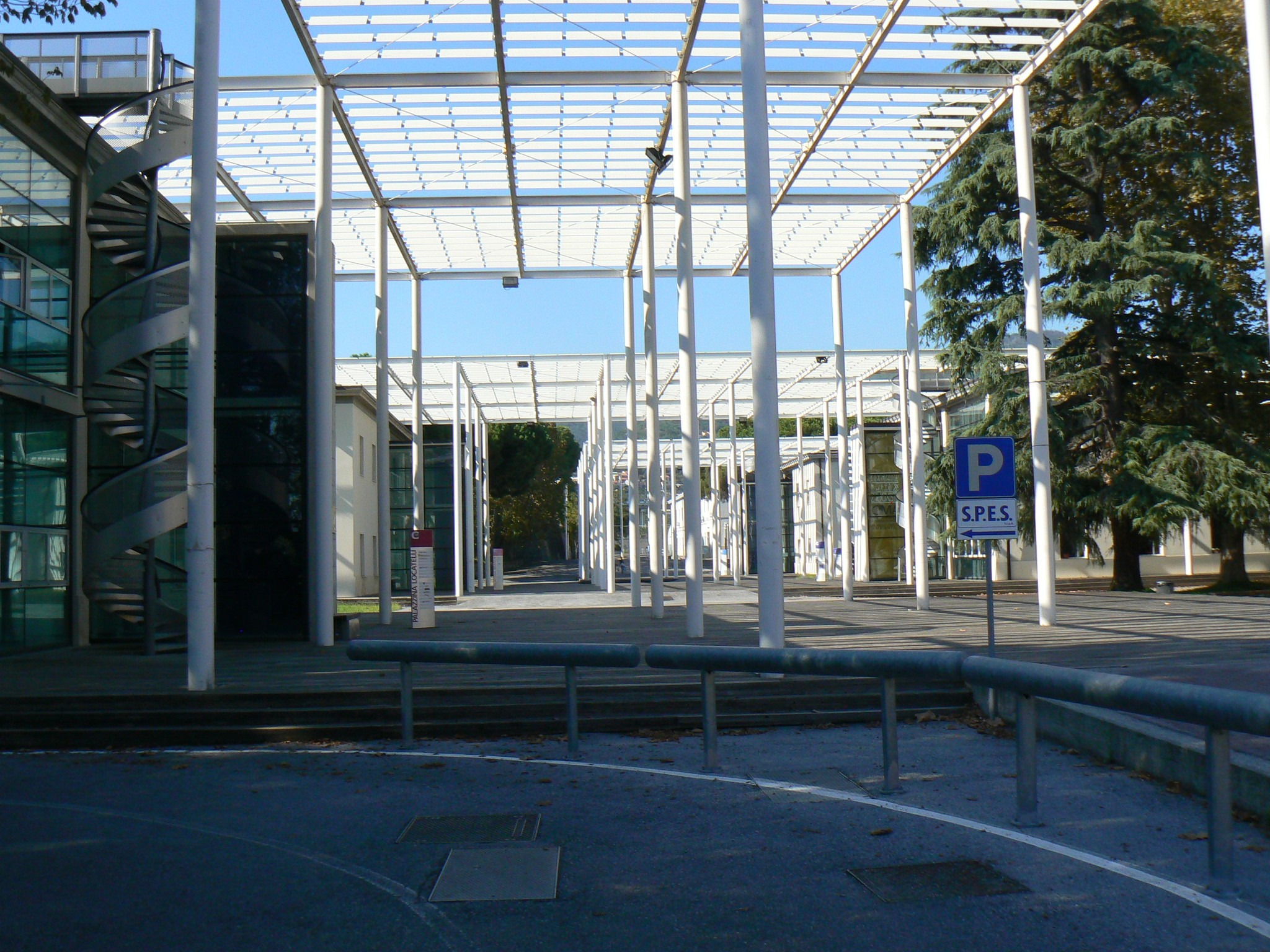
503 276 519 288
517 361 528 368
645 147 673 175
816 357 827 363
